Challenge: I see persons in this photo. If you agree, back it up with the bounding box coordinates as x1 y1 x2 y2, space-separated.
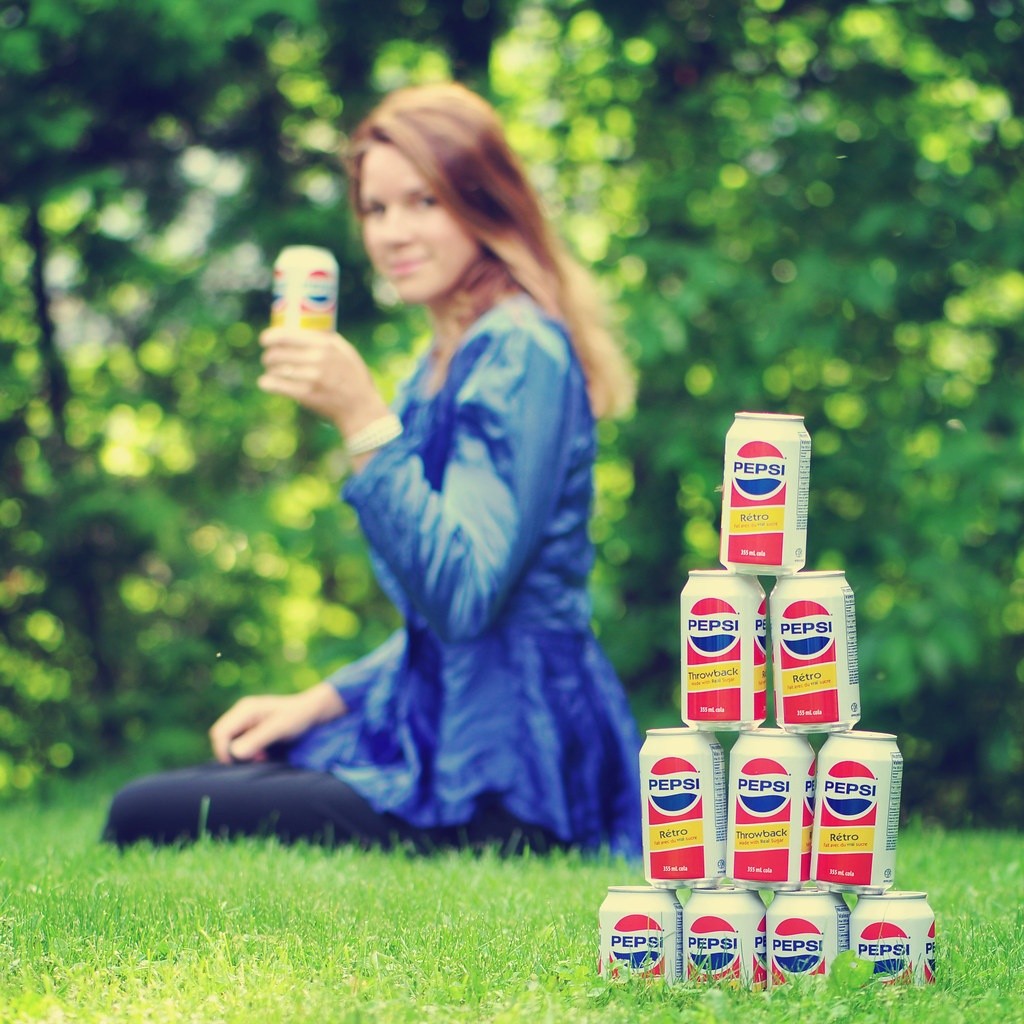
99 81 643 863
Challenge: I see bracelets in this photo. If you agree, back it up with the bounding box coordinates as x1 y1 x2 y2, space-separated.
341 412 403 452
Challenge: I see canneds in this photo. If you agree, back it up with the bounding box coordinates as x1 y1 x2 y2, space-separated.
270 245 339 328
596 410 936 989
